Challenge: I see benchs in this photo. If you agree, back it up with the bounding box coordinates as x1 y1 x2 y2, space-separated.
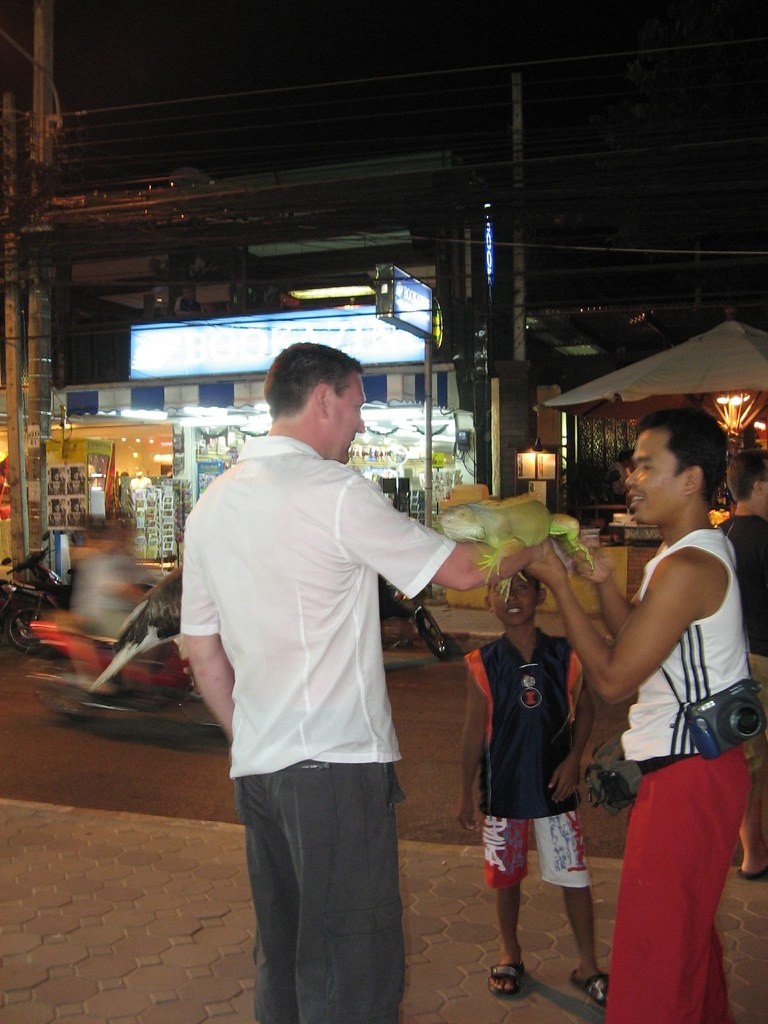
571 504 627 535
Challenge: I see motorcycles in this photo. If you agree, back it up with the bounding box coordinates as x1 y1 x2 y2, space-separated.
379 573 449 659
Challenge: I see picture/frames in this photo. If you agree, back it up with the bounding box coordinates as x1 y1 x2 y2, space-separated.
45 463 87 531
197 461 223 500
516 452 557 507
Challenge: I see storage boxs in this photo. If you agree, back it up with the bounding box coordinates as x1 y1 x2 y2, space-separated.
539 546 628 613
445 582 489 610
439 484 490 511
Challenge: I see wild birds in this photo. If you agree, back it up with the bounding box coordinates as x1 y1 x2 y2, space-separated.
90 565 189 694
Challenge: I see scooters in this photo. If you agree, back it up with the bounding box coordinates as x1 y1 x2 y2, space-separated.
0 531 155 655
26 582 205 719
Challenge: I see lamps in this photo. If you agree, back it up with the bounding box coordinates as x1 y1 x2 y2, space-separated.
289 273 376 300
716 391 751 407
532 437 543 452
177 415 249 427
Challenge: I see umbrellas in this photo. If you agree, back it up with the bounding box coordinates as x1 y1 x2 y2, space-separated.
532 317 768 519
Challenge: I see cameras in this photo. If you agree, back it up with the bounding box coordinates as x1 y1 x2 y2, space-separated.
683 679 767 760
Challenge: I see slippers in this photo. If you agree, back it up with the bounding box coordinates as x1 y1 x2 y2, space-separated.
737 867 768 880
571 968 608 1009
488 959 528 998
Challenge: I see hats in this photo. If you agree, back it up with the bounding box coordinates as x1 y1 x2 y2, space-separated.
619 449 635 462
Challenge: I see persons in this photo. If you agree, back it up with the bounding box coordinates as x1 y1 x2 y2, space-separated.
529 406 751 1024
47 466 86 527
457 573 610 1008
717 449 768 879
181 342 570 1024
130 472 152 493
